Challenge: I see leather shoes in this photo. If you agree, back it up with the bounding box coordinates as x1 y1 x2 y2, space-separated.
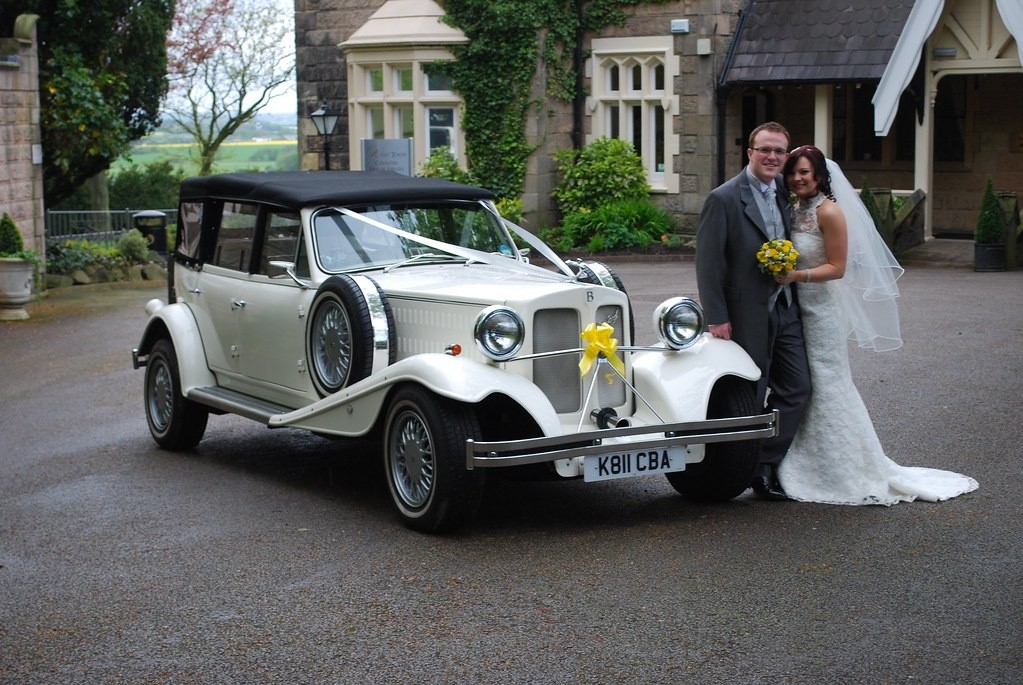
754 476 787 499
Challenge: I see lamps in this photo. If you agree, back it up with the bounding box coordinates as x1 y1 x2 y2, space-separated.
934 47 957 58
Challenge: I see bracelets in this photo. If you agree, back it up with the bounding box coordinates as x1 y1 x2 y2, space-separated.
805 269 811 284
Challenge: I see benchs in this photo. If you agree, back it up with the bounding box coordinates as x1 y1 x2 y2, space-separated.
216 236 306 272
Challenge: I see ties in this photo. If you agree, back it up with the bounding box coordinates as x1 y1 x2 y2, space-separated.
764 188 777 214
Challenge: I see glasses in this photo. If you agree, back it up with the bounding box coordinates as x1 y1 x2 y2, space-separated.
749 147 787 156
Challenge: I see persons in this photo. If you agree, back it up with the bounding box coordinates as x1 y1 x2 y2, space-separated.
775 145 980 507
692 122 812 506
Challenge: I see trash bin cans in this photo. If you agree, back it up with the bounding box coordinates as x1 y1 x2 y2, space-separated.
132 210 171 260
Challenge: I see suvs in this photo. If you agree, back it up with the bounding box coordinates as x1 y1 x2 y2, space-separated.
133 170 778 533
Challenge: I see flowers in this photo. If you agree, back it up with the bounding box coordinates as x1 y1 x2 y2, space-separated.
785 192 798 211
758 238 799 278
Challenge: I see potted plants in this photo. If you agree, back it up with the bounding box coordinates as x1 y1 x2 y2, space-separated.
0 212 34 321
973 178 1008 271
